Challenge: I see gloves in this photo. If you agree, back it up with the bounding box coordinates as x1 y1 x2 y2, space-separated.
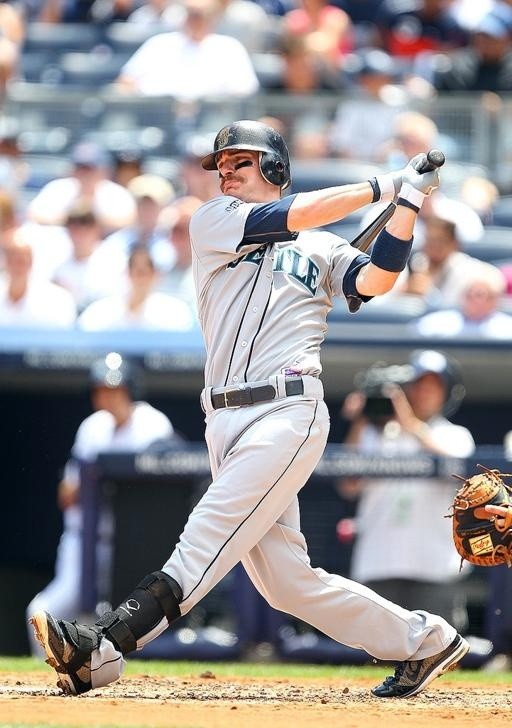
371 152 442 207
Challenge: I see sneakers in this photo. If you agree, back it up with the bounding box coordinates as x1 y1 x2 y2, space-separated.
370 634 471 699
27 609 95 695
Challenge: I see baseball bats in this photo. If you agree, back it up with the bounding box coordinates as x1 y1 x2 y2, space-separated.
346 149 444 251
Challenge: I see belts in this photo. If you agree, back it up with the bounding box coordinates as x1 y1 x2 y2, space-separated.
210 378 305 409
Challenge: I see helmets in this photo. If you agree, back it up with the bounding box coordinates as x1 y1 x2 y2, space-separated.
200 119 292 187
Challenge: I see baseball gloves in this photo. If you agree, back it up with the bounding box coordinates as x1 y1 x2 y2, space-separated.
443 463 509 573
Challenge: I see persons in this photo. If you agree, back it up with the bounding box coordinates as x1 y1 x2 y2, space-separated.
1 0 511 352
24 344 178 669
22 115 472 700
323 344 480 639
449 466 512 573
